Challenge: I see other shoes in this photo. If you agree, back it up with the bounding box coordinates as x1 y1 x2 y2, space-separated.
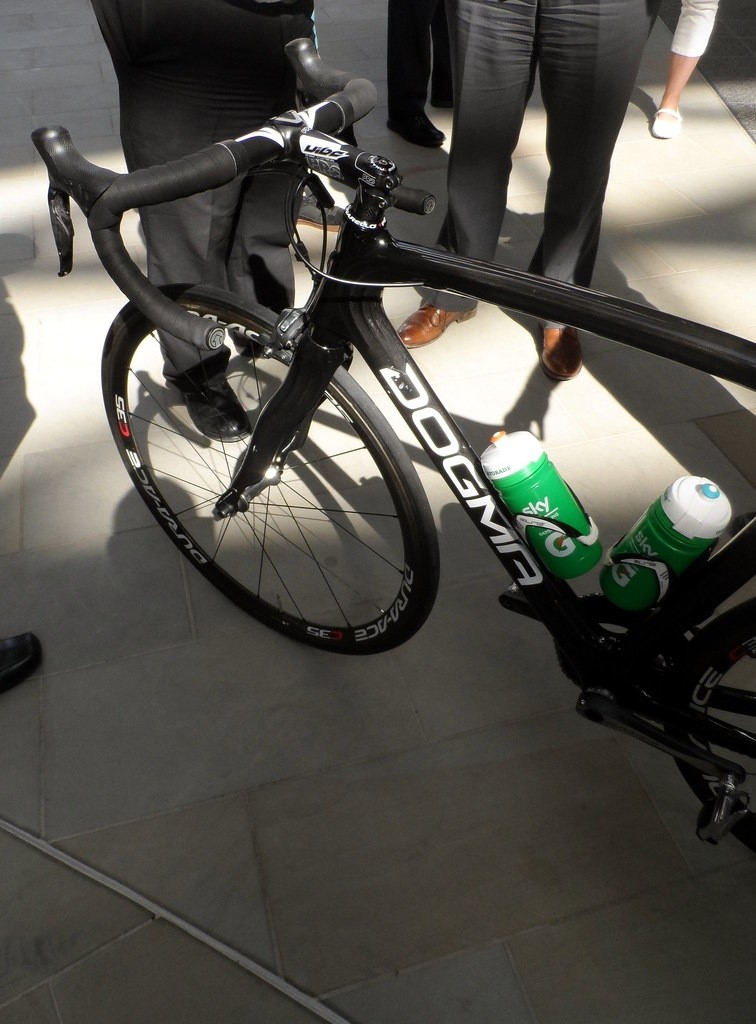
430 90 456 108
387 118 445 148
0 631 41 694
652 108 683 139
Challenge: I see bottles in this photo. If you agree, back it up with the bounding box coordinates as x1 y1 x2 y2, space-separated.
597 476 733 613
479 429 602 581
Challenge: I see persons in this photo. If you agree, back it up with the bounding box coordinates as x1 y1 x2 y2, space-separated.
87 0 719 444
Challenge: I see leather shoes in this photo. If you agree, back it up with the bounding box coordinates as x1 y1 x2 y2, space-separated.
397 302 475 348
182 379 252 442
537 320 583 381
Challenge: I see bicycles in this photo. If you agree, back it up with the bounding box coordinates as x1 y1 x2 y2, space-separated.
31 39 756 854
